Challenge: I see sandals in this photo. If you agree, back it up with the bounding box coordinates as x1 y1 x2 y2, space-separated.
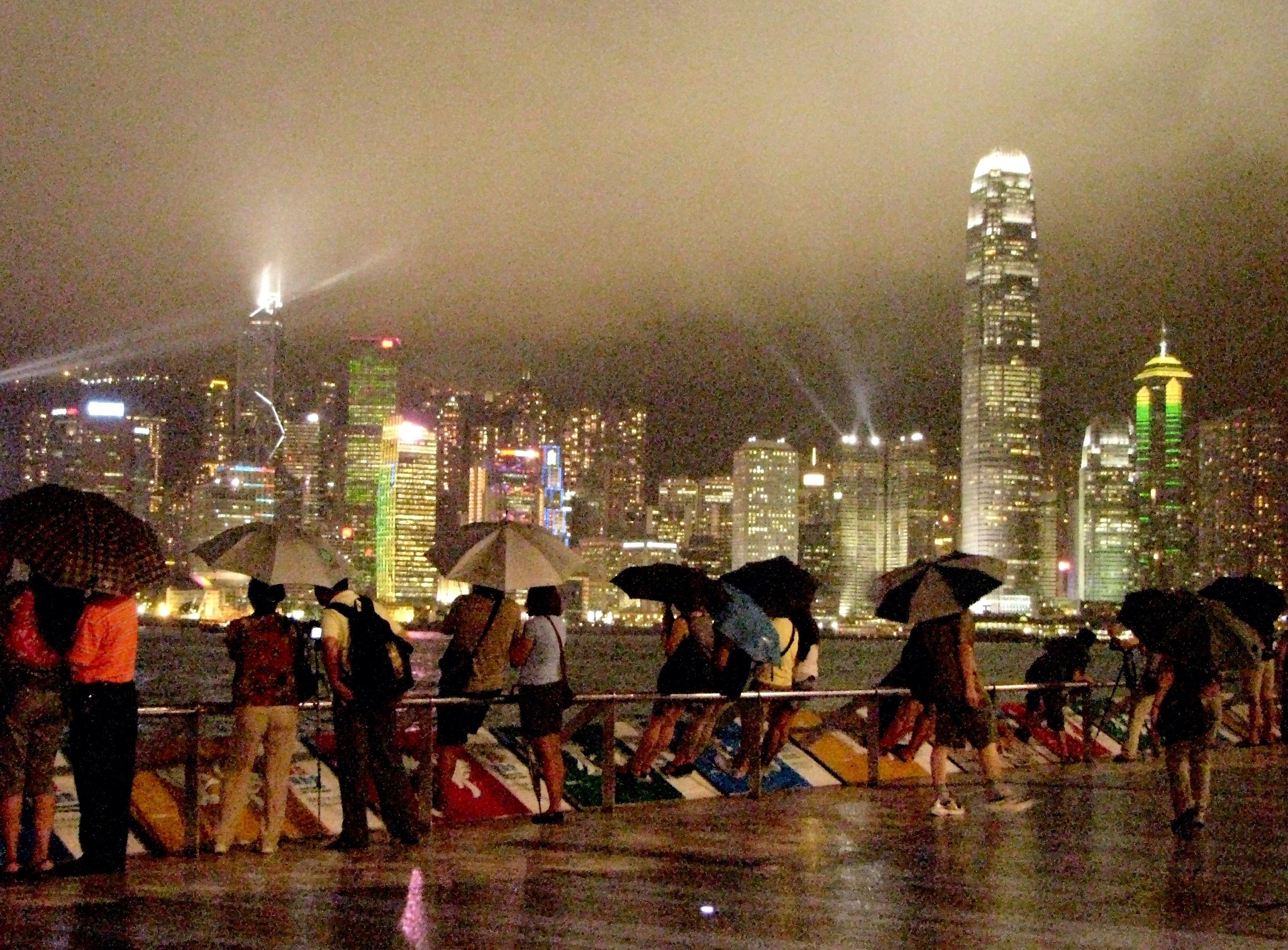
0 859 56 886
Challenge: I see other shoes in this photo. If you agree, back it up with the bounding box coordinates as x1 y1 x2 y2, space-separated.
890 743 917 762
986 793 1036 812
57 857 127 878
212 844 229 854
260 847 279 854
1169 806 1208 834
529 810 566 825
1234 737 1274 748
390 833 419 845
931 799 967 817
325 833 371 852
618 757 772 781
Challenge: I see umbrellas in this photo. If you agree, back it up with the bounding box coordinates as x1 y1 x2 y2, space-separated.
608 561 735 614
189 522 358 589
1116 576 1288 673
423 510 592 593
714 583 782 668
718 555 818 613
0 484 170 605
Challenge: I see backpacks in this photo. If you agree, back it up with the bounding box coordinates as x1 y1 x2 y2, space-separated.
902 619 961 707
322 594 416 706
656 614 717 698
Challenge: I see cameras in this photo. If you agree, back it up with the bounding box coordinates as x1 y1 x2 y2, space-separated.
301 620 323 639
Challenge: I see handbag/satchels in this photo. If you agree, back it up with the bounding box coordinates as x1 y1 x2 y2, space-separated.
562 678 573 710
289 616 318 703
435 640 474 691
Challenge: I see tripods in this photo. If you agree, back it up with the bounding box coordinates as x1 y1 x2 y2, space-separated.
1087 650 1150 761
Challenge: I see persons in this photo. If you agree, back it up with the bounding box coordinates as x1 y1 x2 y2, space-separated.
0 573 64 883
617 599 818 783
870 550 1007 624
508 585 567 823
315 577 430 851
834 620 936 762
931 612 1038 815
1013 627 1106 764
1111 621 1222 843
56 593 139 879
1239 651 1279 746
210 577 321 854
409 584 521 819
